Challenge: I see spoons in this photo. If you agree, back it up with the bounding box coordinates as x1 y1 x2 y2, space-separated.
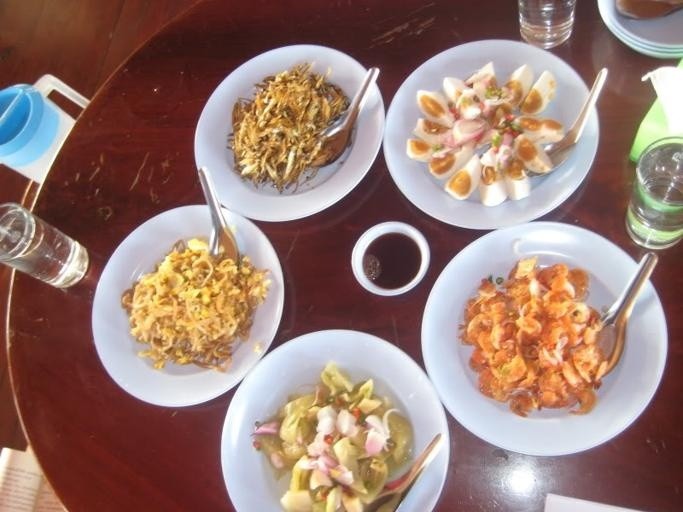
362 434 442 510
195 167 243 264
317 65 381 161
524 66 609 179
598 252 659 376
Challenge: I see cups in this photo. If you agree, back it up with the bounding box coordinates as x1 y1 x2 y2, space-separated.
1 200 88 290
624 121 683 255
515 2 576 50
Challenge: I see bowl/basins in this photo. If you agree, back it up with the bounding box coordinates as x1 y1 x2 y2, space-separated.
351 220 433 297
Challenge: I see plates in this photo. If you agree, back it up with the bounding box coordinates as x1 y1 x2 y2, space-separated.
381 40 603 232
420 221 672 460
88 205 288 409
192 42 386 223
594 1 683 61
219 329 452 511
